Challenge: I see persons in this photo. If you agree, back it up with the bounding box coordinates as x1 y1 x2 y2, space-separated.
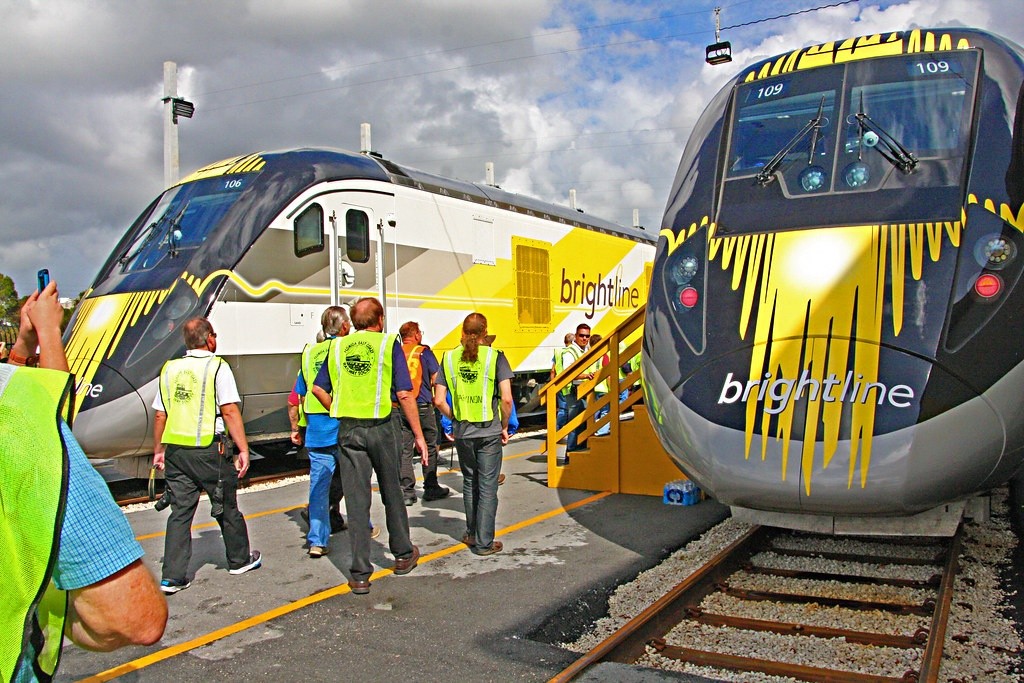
150 316 262 592
550 323 641 465
311 297 429 594
0 363 169 683
434 312 516 556
0 280 70 373
287 297 519 594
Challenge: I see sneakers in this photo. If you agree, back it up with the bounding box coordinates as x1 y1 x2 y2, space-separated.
229 550 261 575
309 546 329 555
404 495 417 505
423 485 449 499
370 527 380 538
160 577 191 592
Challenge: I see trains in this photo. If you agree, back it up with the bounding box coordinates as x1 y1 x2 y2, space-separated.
642 28 1023 537
61 145 659 484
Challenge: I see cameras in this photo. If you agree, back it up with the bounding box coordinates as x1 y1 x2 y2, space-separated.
154 487 173 512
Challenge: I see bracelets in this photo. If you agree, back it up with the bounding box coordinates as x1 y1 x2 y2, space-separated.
8 349 39 366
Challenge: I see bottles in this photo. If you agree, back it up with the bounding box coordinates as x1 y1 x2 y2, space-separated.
662 478 702 505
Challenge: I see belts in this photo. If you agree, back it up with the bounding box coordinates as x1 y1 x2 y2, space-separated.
214 434 221 442
416 404 429 408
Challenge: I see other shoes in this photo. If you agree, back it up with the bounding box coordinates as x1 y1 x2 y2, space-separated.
332 523 348 532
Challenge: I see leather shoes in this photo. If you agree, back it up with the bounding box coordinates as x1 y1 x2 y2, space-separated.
462 532 475 545
478 542 503 555
498 474 506 484
395 545 420 573
349 576 369 593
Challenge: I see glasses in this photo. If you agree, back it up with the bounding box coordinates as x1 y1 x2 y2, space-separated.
418 330 424 336
579 334 591 338
212 333 217 338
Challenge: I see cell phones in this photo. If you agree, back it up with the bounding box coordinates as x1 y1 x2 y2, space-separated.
37 269 49 295
